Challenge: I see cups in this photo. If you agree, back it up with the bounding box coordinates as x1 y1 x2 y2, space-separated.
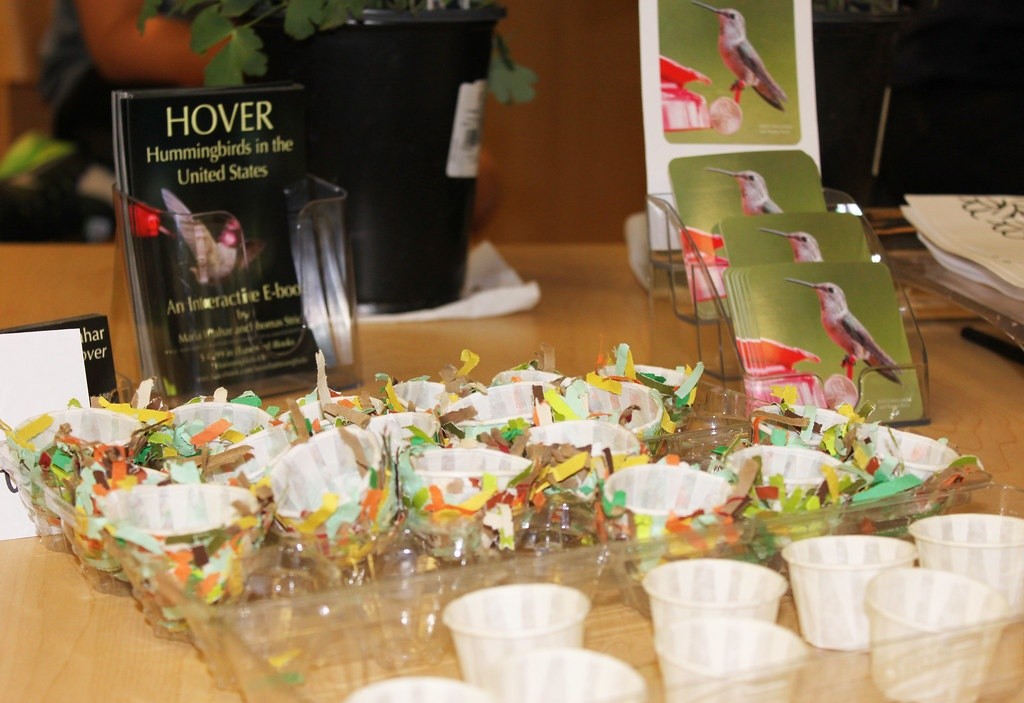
24 361 1024 703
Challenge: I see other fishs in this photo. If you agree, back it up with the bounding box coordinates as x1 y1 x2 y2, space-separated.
690 1 787 112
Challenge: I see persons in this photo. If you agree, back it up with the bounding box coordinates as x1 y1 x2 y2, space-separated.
38 63 176 242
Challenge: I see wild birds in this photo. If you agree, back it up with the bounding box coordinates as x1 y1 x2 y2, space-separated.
706 168 785 213
758 228 824 262
785 276 901 387
